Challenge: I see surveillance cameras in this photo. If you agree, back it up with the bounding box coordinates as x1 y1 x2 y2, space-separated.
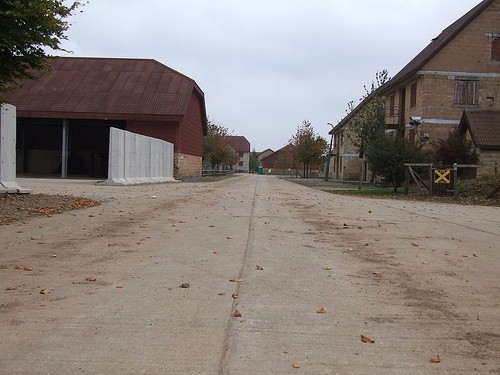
410 116 422 121
